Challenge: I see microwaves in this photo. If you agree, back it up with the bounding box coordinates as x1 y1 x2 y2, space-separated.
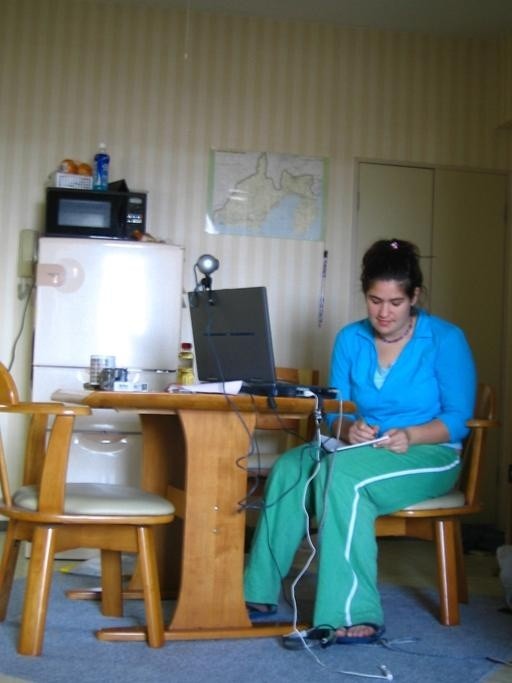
44 186 148 242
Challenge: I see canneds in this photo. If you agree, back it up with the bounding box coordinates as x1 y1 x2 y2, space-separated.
99 366 128 391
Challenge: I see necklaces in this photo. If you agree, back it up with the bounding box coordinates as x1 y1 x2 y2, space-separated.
378 314 414 345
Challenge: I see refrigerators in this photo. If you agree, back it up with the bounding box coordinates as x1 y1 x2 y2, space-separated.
25 237 185 559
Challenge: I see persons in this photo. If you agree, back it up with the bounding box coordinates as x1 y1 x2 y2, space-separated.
239 235 478 644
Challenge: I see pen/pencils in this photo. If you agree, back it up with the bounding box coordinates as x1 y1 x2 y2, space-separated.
358 414 378 438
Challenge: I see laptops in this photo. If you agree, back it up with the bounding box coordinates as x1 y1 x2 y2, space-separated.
188 285 337 399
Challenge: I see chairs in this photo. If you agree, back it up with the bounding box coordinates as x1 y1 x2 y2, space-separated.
0 362 177 656
247 365 501 626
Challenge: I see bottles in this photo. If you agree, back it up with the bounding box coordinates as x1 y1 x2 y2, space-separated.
176 343 195 391
91 142 112 190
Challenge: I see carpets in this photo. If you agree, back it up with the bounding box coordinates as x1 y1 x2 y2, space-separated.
0 565 512 683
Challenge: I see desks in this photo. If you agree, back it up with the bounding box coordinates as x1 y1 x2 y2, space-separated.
49 390 356 642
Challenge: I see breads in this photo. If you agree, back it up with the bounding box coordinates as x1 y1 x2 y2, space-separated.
62 160 93 176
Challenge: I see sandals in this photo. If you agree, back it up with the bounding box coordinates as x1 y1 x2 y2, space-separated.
246 603 275 618
337 623 386 644
